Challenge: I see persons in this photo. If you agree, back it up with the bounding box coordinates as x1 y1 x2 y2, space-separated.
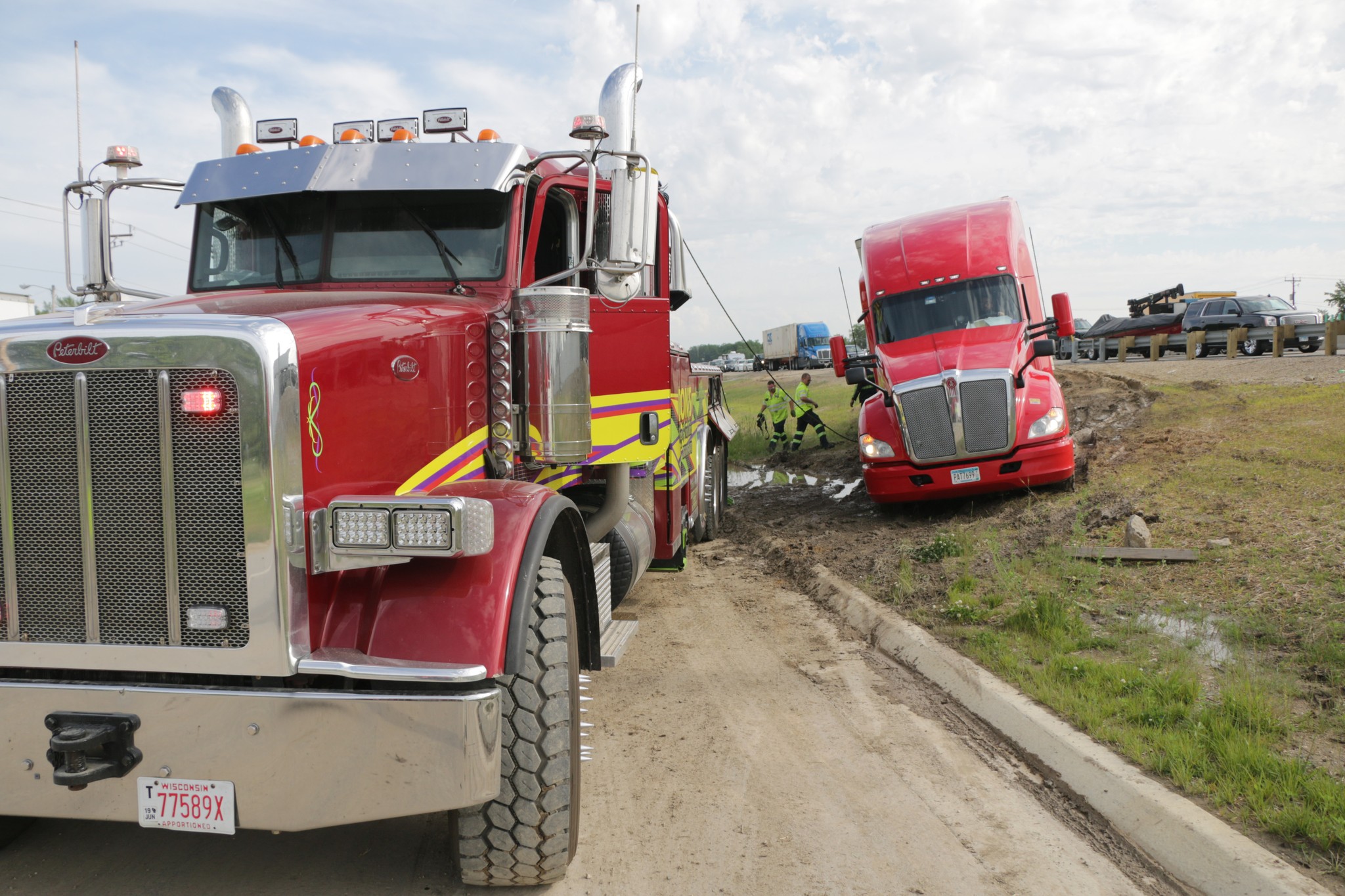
757 381 796 453
979 293 1005 318
791 373 834 452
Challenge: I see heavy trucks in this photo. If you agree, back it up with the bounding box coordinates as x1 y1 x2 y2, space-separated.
762 321 834 371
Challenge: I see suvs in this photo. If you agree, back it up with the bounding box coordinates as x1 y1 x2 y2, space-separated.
752 353 769 371
1180 295 1325 358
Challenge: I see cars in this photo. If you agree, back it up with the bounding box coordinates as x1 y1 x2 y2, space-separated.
733 359 746 372
694 359 735 373
742 358 754 372
845 344 868 363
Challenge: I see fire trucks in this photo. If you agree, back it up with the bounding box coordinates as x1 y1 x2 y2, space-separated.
829 193 1080 509
0 26 741 891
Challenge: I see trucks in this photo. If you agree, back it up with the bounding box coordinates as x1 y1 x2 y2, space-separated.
1043 318 1094 360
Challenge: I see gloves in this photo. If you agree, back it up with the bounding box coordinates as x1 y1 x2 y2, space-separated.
790 410 796 417
757 411 762 418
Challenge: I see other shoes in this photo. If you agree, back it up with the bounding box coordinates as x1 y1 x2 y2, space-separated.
821 444 835 449
766 446 773 453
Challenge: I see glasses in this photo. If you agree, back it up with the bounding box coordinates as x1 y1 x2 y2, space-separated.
768 386 775 391
803 379 811 382
